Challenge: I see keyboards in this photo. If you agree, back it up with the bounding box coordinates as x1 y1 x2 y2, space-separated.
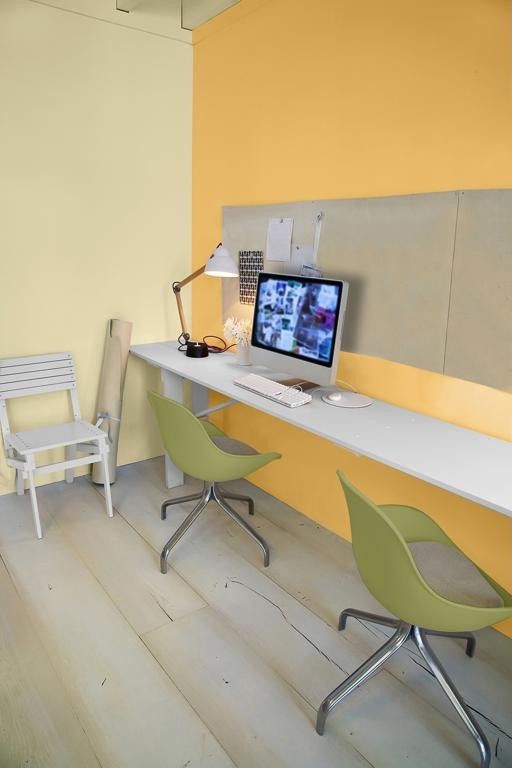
233 369 312 408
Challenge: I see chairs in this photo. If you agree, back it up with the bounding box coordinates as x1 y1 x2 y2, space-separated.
146 391 281 573
0 352 113 539
316 469 511 767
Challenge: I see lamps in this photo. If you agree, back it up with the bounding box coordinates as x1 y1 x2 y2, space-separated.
173 242 239 358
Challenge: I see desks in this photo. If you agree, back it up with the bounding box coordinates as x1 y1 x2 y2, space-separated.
128 338 512 518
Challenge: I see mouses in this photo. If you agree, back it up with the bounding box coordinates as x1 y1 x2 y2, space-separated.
330 391 341 400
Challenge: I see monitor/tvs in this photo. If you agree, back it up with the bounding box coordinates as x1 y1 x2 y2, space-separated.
249 269 352 388
